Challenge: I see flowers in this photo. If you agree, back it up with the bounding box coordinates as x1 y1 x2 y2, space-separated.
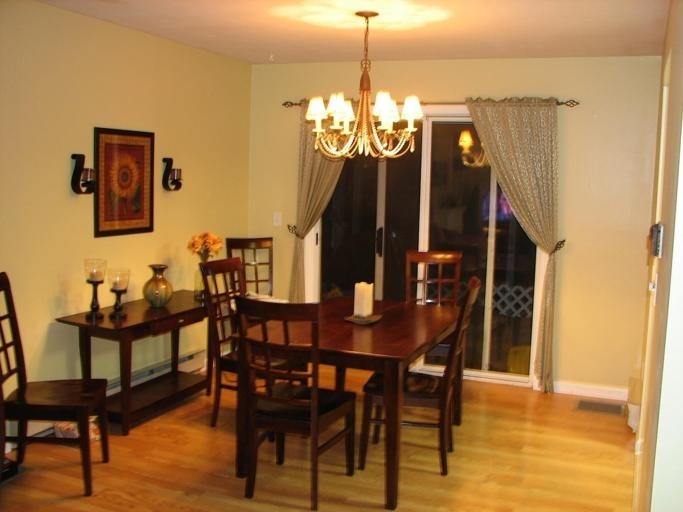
186 232 224 280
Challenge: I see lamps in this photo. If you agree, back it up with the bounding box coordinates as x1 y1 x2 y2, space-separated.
161 157 182 191
456 130 490 168
304 10 424 161
69 153 96 194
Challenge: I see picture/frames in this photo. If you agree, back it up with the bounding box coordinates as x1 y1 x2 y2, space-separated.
92 126 154 237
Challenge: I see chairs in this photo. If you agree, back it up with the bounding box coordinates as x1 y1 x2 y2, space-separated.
404 249 463 348
357 277 482 477
225 237 275 324
199 256 310 428
0 272 110 497
232 294 356 511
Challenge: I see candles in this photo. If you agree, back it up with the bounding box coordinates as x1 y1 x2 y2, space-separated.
89 272 103 282
353 282 373 317
113 280 125 290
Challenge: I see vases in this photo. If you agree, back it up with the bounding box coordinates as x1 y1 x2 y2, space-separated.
142 264 173 308
193 256 212 302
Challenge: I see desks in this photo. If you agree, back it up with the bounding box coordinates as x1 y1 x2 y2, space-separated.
54 289 219 436
226 298 472 510
